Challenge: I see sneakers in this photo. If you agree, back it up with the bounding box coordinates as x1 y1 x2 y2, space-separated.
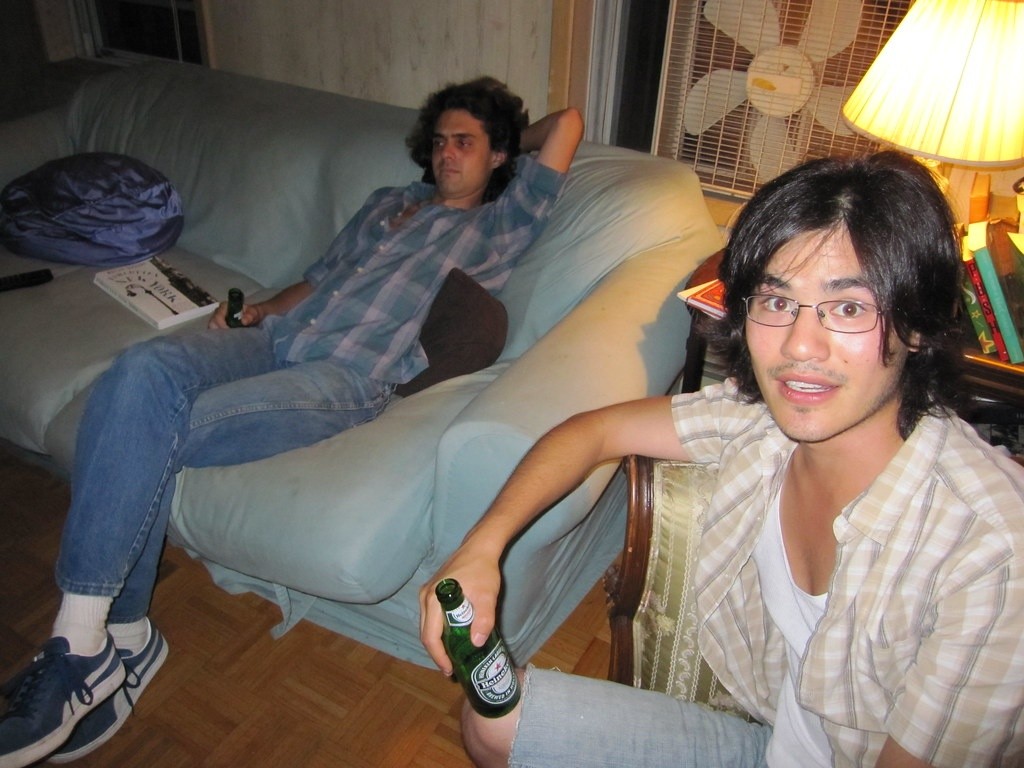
45 615 170 764
0 630 127 767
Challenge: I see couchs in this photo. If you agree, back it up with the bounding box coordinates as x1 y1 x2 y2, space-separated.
0 50 725 676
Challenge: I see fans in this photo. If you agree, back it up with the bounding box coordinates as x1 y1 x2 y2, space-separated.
651 0 917 201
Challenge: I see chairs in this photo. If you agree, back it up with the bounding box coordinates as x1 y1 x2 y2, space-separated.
603 455 763 725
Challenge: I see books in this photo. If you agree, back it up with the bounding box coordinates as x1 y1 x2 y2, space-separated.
952 220 1024 365
93 256 220 330
969 173 1024 234
677 279 727 320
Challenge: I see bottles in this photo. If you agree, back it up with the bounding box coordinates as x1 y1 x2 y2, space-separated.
225 288 244 328
436 579 521 718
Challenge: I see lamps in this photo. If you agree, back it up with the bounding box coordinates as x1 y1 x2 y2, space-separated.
840 0 1024 226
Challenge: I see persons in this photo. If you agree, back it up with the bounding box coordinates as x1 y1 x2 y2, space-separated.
419 151 1023 767
0 76 583 768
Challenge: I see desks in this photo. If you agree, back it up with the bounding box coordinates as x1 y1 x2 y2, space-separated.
677 276 1024 470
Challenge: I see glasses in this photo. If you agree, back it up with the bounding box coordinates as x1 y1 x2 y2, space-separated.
741 294 908 333
